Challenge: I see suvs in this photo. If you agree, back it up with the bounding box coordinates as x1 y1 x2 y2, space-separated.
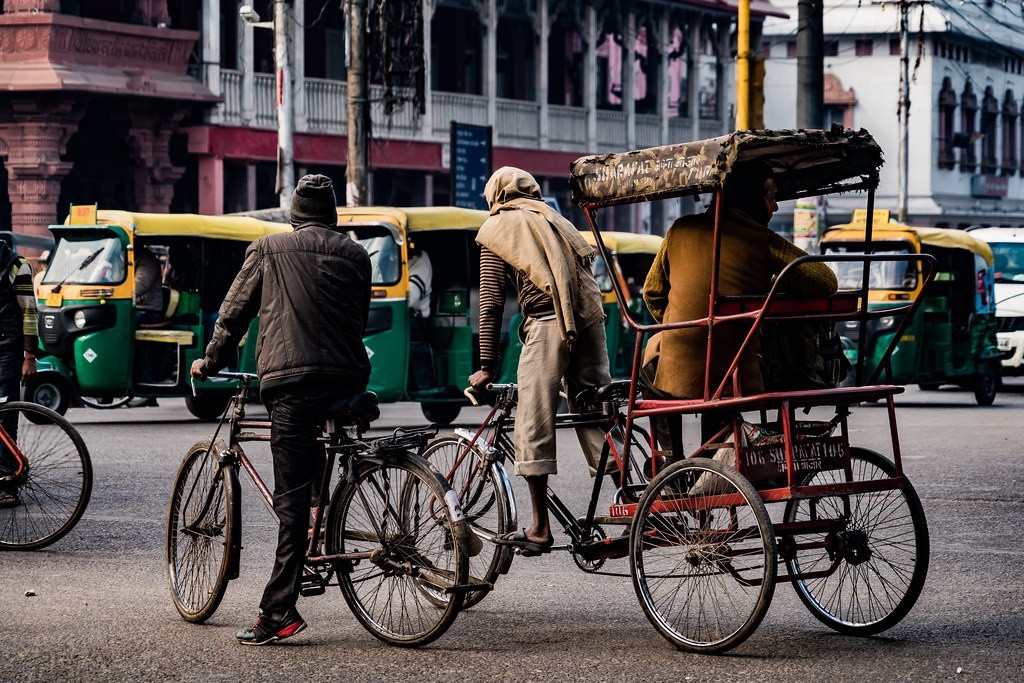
917 224 1024 390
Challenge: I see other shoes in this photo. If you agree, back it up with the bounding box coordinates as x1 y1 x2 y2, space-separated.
644 458 687 494
0 491 16 508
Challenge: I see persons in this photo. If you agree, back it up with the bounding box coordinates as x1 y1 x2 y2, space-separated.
191 173 372 644
392 233 432 392
33 243 191 409
468 166 655 554
0 240 39 508
642 162 839 495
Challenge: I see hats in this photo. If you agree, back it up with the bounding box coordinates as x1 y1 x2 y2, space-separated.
290 174 338 230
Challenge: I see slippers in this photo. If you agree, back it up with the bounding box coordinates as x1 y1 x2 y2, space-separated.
489 527 552 554
608 530 652 559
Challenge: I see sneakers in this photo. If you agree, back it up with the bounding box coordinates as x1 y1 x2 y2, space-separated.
235 606 308 646
309 506 327 529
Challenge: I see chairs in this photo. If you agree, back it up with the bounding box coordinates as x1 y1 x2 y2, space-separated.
140 285 180 327
633 291 857 413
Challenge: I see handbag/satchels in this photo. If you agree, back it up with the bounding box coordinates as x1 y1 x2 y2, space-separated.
758 318 857 393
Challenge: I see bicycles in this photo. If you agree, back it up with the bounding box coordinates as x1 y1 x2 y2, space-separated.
0 396 94 551
164 372 493 649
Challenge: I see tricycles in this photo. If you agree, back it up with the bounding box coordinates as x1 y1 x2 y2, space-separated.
395 128 929 656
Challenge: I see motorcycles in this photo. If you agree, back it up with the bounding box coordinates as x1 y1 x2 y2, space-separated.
818 211 1017 407
0 205 666 424
